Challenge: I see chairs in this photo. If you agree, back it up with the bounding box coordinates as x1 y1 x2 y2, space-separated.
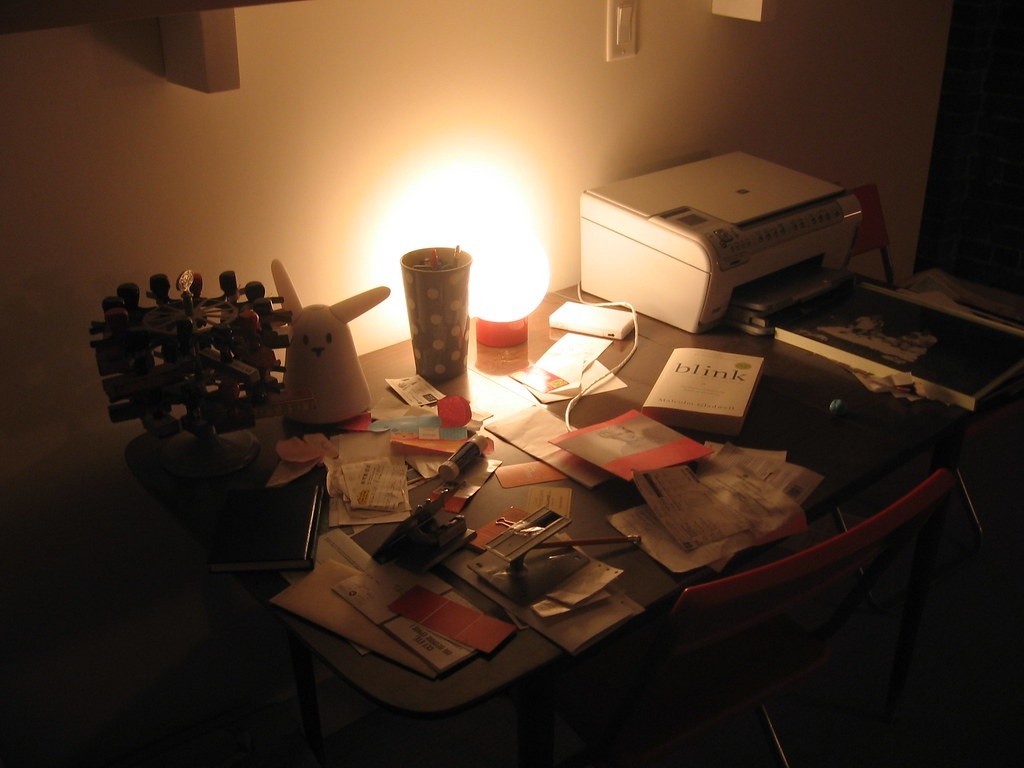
553 468 956 767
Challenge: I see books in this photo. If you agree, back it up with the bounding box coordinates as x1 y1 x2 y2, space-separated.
773 284 1024 411
640 348 766 438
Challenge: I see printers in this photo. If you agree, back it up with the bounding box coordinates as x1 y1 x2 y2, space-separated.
580 150 862 335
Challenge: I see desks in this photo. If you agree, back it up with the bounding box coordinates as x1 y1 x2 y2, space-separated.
125 271 1024 768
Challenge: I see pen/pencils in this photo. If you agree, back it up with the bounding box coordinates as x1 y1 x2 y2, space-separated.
534 534 641 549
413 245 462 271
